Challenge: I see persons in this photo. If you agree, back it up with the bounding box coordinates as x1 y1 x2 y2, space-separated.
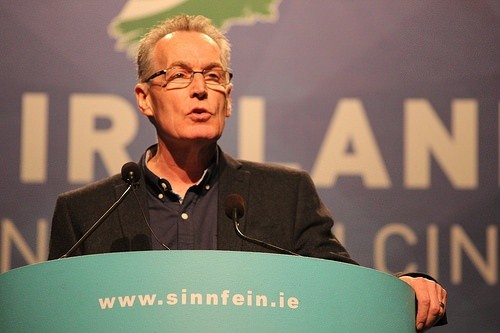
46 14 448 330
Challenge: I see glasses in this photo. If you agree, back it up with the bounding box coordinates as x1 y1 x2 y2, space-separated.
144 70 231 95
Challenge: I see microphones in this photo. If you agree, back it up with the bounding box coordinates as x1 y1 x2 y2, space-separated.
224 193 300 255
60 162 141 258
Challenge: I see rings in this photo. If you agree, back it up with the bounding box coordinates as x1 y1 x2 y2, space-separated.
439 302 444 308
